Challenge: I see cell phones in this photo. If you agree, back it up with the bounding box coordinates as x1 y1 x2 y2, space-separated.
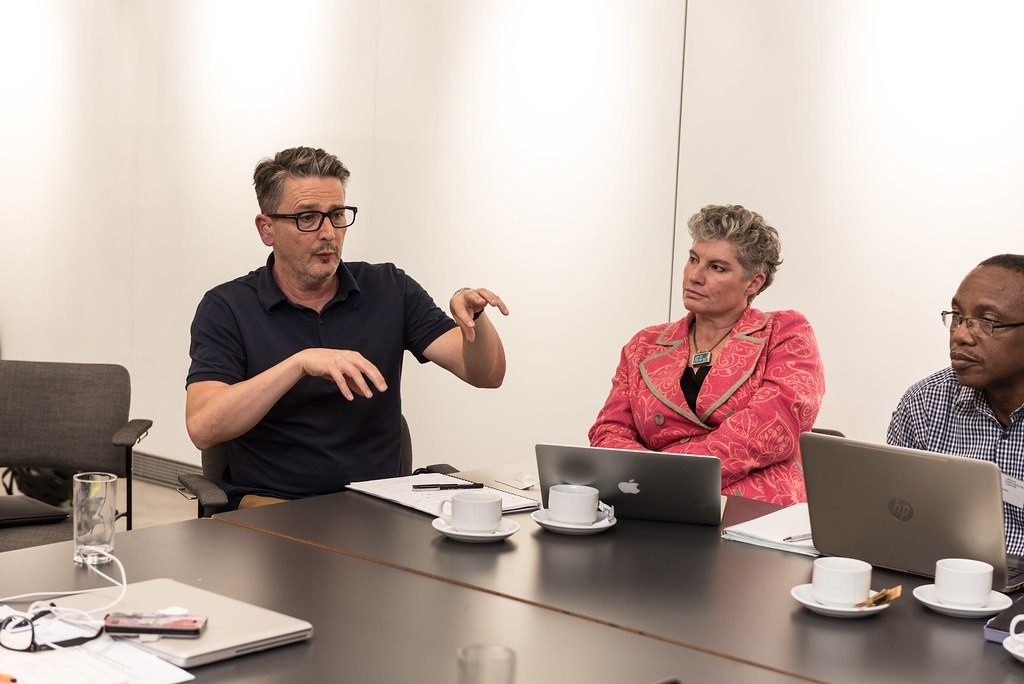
104 612 208 637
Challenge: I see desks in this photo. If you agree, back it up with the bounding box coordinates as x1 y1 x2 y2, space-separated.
0 486 1024 684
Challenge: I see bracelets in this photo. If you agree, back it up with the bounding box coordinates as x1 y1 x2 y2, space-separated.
455 288 484 320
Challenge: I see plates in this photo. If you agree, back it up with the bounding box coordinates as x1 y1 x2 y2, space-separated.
792 584 889 617
531 507 616 534
1002 634 1024 664
432 514 519 542
912 583 1012 618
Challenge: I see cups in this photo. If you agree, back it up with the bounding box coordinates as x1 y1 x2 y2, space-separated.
812 558 873 607
1009 613 1024 653
549 485 599 525
73 473 118 565
438 494 501 534
934 558 994 608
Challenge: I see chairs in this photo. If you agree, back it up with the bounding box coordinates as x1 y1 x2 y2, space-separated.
1 360 154 548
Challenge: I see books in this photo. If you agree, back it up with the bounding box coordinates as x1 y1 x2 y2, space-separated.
720 503 821 558
344 472 542 517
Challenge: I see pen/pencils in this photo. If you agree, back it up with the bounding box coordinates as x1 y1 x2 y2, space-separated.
412 483 484 490
783 533 812 542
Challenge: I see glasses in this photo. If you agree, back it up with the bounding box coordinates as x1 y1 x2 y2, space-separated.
942 311 1024 338
265 205 357 232
0 602 110 653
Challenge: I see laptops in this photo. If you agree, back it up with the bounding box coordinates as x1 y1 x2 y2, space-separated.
798 433 1024 591
535 445 728 525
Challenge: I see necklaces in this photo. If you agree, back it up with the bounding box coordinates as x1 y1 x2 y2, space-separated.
692 325 734 367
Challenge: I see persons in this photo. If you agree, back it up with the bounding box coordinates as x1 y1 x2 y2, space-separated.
586 204 825 507
184 147 510 519
884 248 1024 557
